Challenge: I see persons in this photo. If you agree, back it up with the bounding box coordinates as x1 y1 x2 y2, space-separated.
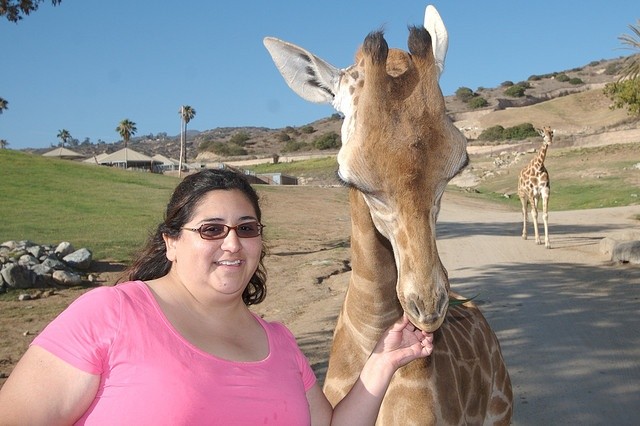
0 168 436 425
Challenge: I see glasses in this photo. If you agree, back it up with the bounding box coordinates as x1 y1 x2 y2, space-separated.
179 221 266 240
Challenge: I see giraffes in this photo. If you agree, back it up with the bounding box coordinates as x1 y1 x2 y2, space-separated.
517 126 554 249
262 4 514 426
